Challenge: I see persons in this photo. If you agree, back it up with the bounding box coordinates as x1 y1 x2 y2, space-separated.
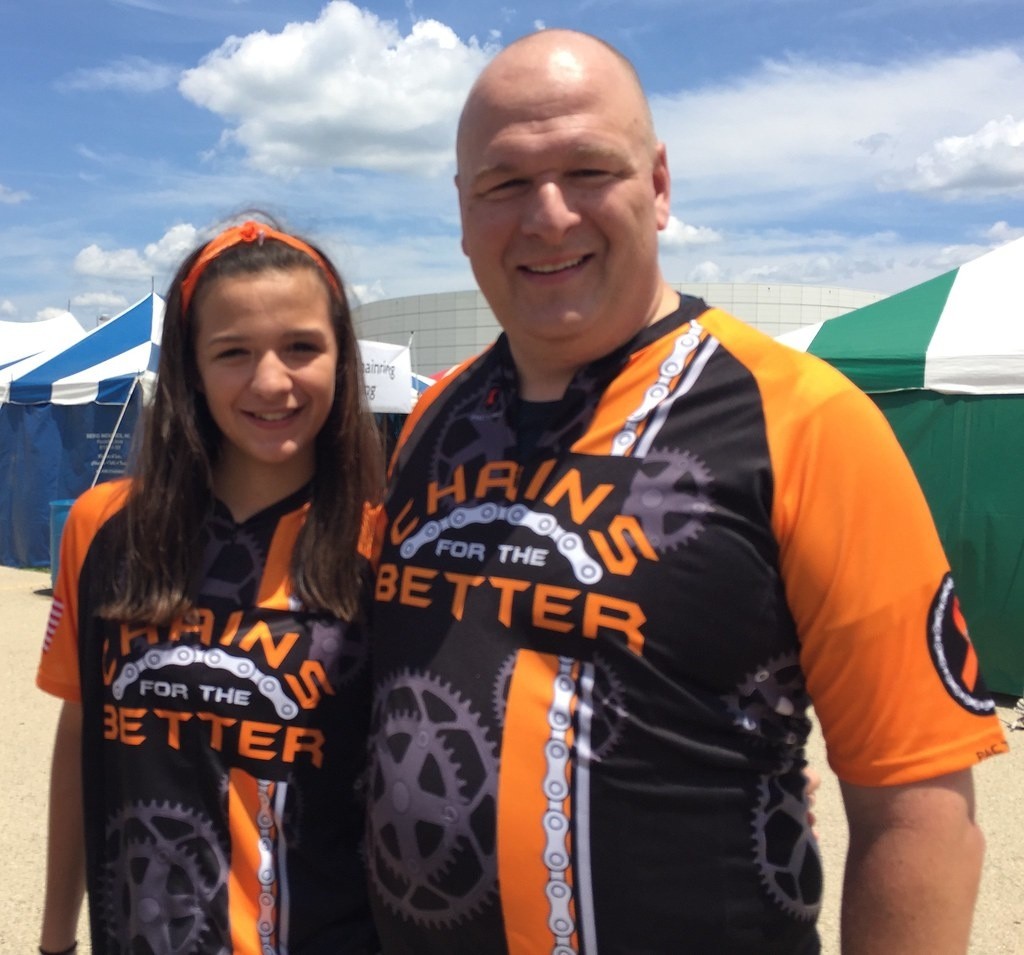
362 29 1009 955
35 223 388 955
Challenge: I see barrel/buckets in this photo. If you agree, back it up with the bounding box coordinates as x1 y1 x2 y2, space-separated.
49 499 77 589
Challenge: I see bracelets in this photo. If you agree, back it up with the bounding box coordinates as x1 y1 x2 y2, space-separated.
39 942 78 955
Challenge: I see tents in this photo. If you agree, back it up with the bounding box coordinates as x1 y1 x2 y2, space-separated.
1 234 1023 698
